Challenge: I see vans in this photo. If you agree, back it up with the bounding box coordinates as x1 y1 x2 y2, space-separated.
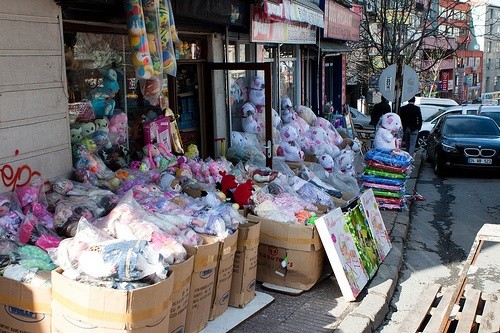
417 104 500 148
413 97 459 121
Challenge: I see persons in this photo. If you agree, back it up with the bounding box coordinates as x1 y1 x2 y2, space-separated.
0 153 237 281
371 95 391 133
399 96 422 158
250 163 358 229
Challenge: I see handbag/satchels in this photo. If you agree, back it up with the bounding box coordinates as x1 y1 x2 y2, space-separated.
68 71 96 121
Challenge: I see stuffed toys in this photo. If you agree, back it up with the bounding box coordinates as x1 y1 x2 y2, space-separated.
231 76 343 162
69 69 128 143
316 149 355 176
374 112 403 150
143 143 279 211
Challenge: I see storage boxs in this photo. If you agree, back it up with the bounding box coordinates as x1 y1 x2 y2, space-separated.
0 192 356 333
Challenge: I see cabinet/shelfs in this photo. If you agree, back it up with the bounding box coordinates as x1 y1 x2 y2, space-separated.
168 59 206 161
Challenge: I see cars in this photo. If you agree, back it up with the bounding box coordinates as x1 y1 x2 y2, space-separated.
350 107 375 130
427 115 500 177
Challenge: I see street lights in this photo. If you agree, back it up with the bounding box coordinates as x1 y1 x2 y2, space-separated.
445 35 458 96
